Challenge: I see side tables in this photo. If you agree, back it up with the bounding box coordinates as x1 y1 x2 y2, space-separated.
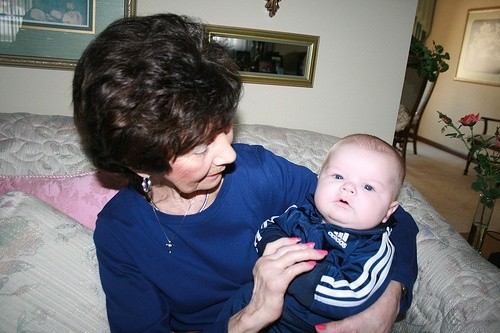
459 231 500 262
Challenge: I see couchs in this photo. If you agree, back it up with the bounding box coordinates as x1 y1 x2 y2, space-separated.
0 112 500 333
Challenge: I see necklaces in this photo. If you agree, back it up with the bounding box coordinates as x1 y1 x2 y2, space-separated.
147 189 210 254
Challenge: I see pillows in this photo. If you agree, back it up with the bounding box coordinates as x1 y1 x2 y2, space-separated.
0 190 112 333
0 170 120 232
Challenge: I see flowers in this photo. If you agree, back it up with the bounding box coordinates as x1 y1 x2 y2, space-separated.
435 110 500 247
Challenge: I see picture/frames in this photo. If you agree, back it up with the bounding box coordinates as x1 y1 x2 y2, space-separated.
454 6 500 87
0 0 137 72
201 23 320 88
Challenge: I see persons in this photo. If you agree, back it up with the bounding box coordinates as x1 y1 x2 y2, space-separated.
72 14 419 333
216 134 406 333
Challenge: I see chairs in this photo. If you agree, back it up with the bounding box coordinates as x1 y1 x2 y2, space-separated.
463 117 500 176
392 63 440 162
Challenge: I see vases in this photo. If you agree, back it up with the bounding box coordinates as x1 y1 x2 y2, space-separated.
465 193 497 255
